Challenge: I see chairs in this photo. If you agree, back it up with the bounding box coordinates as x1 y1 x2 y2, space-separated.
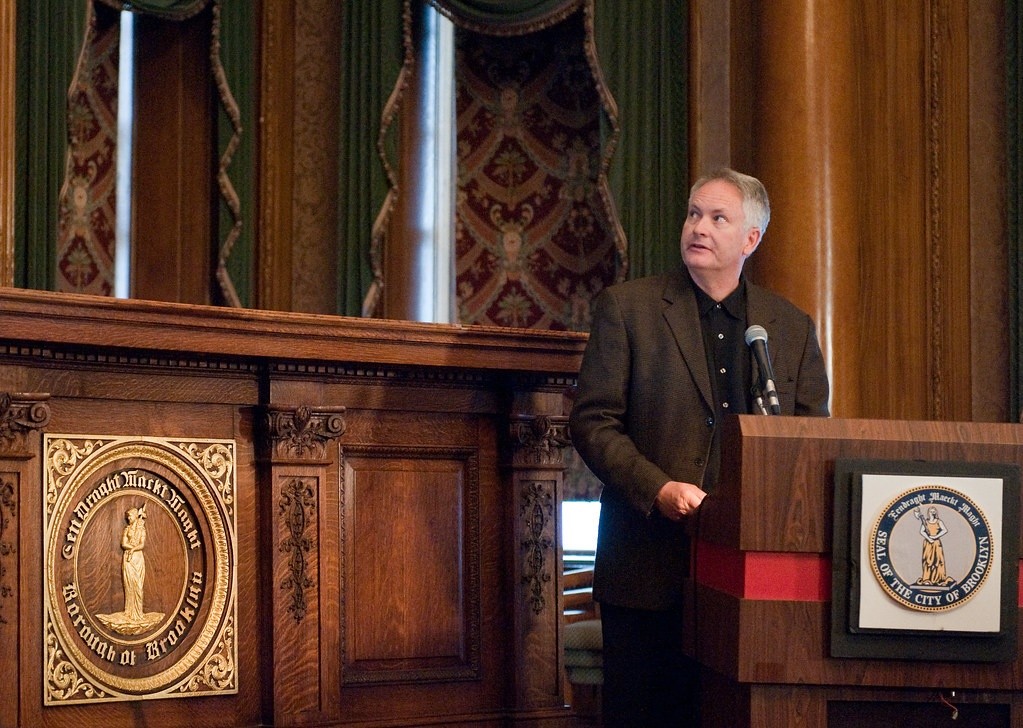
560 568 603 722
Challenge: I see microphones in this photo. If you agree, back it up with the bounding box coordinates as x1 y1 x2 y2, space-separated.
744 325 782 415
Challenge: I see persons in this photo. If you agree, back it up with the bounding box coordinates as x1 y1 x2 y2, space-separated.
570 167 827 728
